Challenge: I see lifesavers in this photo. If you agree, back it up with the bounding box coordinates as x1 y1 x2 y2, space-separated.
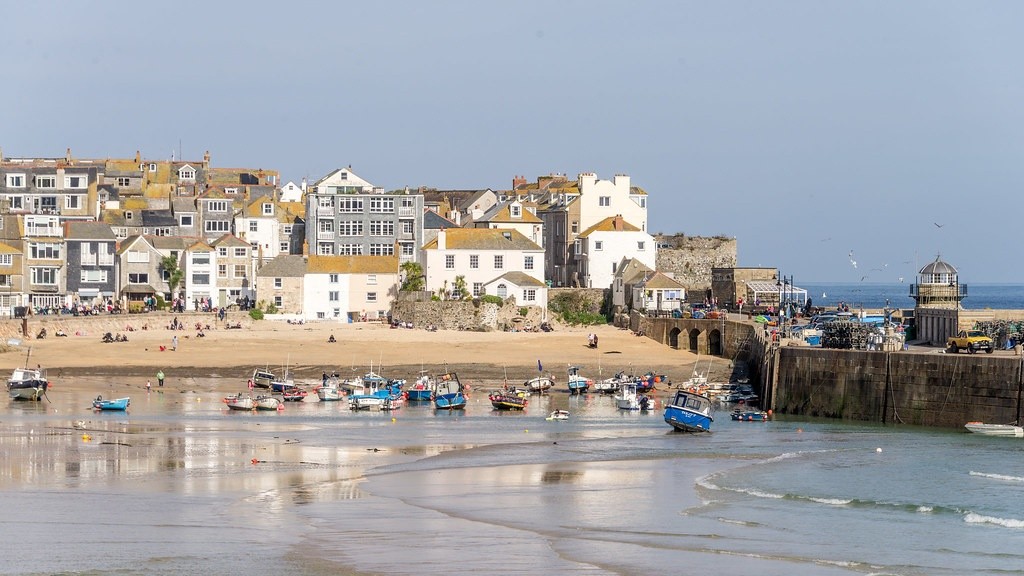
248 380 253 387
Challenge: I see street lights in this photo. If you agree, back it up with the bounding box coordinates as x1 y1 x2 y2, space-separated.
784 275 794 319
771 271 781 326
775 275 786 338
947 274 960 336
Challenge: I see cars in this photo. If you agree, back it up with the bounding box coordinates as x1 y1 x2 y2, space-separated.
790 310 885 342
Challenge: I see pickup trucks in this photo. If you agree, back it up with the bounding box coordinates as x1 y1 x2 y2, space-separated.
947 329 995 354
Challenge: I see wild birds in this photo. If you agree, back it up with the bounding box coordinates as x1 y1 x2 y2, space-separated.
823 250 911 298
934 223 945 228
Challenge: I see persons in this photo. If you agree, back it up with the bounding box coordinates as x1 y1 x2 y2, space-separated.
126 323 148 332
588 334 598 348
173 336 178 350
703 296 719 311
328 334 336 343
837 301 849 312
143 295 158 312
778 298 803 325
34 299 122 316
195 322 210 337
158 370 165 386
503 322 554 333
165 317 185 331
390 319 435 331
56 329 66 336
322 371 331 386
237 322 241 327
36 329 46 339
145 380 151 390
103 333 128 343
172 295 255 313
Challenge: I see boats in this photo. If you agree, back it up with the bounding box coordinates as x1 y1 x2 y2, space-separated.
565 352 760 410
729 407 772 422
282 386 308 402
253 395 282 412
522 359 553 394
429 360 467 410
251 362 276 387
660 351 715 432
222 391 255 410
546 408 571 422
314 349 436 411
92 395 130 411
270 352 296 391
486 367 529 411
4 345 50 402
